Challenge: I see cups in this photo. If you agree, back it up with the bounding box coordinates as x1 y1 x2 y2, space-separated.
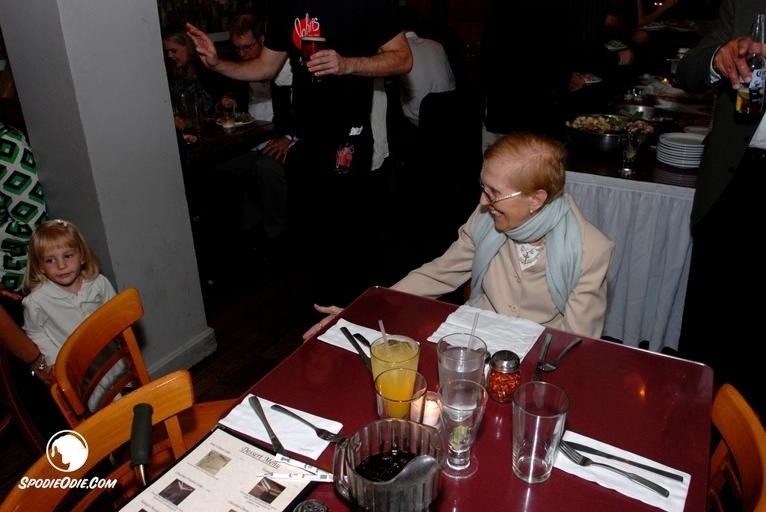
437 378 489 480
436 332 488 396
221 99 235 134
511 380 572 484
371 368 427 425
298 35 325 80
332 416 446 510
369 334 423 425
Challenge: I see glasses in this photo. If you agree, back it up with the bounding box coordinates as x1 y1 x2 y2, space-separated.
235 39 254 51
475 185 532 203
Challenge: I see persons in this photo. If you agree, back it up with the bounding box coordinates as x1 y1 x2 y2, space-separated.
675 0 765 427
19 218 137 411
0 304 56 389
158 2 455 300
456 1 705 148
300 133 616 350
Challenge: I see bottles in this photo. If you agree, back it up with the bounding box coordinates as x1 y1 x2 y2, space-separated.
489 351 520 404
734 13 764 118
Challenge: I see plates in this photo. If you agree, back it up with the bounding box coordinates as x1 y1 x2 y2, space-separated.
655 131 711 171
215 114 254 128
582 17 696 85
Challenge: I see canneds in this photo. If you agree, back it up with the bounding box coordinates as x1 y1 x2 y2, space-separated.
487 348 522 403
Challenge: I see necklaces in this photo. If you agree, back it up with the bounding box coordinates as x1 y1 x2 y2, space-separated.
520 241 540 264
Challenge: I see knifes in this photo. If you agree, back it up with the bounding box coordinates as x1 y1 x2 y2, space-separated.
249 394 284 458
339 325 371 368
535 333 553 380
565 440 686 486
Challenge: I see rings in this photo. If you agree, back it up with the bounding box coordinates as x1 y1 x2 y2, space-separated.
317 322 324 330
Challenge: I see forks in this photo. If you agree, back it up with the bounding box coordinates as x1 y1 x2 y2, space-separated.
536 335 583 373
272 403 346 443
559 439 670 499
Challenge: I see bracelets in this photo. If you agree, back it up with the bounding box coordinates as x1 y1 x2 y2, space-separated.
29 353 48 377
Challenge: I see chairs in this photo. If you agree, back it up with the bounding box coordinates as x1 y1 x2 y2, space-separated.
707 384 766 511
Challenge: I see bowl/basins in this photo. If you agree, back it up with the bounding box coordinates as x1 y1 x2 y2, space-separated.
561 100 673 152
